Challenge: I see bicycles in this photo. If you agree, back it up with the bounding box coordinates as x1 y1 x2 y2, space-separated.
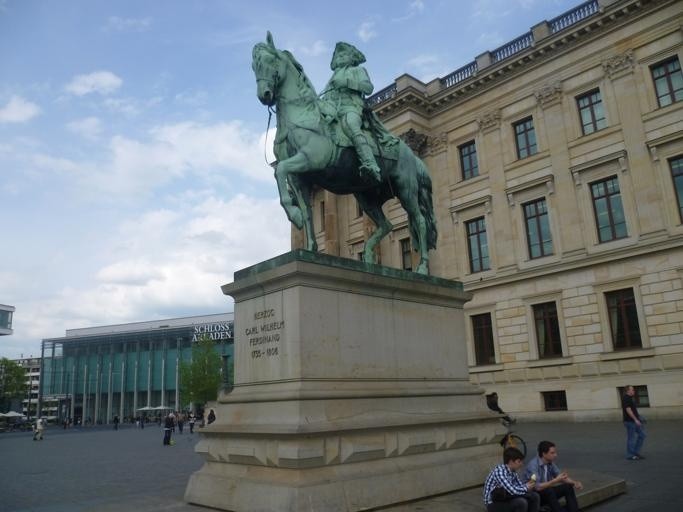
500 416 527 461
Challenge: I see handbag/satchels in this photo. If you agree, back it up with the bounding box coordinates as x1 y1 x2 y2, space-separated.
492 487 515 503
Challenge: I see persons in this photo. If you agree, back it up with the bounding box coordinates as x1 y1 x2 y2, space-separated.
32 418 48 440
162 414 174 446
622 386 646 460
128 412 195 433
207 409 215 424
483 447 540 512
4 417 24 430
523 440 583 512
113 414 119 430
325 41 382 185
63 416 91 429
486 392 516 445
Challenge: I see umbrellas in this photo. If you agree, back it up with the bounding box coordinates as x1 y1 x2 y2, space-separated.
0 411 26 418
138 405 173 418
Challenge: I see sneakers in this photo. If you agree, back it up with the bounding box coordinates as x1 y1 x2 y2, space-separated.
626 454 646 460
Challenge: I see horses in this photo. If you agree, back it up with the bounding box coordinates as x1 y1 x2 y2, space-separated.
250 29 438 275
